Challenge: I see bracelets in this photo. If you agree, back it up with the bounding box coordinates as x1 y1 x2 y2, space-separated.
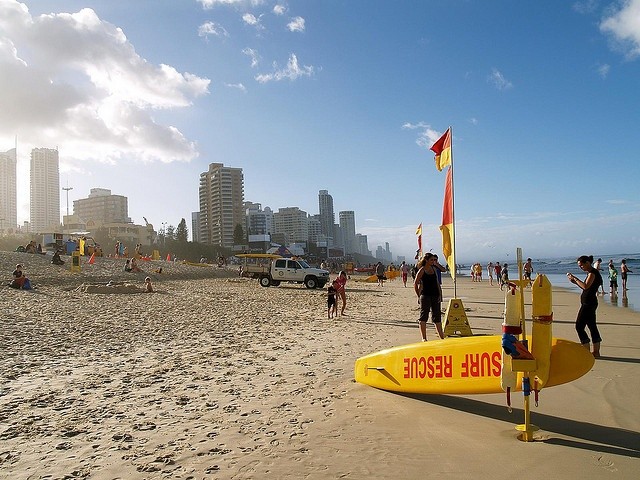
575 277 579 281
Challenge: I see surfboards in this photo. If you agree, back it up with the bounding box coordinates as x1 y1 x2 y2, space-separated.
355 334 595 394
530 275 553 407
235 254 283 258
354 268 373 271
366 271 400 282
501 289 521 413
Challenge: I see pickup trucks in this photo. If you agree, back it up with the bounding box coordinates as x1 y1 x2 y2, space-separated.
238 256 330 288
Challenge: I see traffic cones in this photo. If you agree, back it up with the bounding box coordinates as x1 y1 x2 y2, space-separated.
89 253 95 264
166 254 171 262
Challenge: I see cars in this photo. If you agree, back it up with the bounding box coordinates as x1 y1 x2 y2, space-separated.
354 264 374 272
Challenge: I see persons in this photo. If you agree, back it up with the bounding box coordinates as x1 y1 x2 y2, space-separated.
134 244 143 258
414 252 445 342
608 259 613 268
114 241 128 260
51 250 65 264
523 258 533 286
124 258 142 273
327 280 338 319
375 262 384 287
470 263 482 281
567 254 603 357
610 264 618 294
13 263 27 287
390 262 398 283
26 240 42 253
498 262 509 291
495 262 502 283
215 256 226 269
432 255 446 302
145 277 153 292
621 259 633 289
400 261 409 287
321 260 326 269
334 270 347 317
487 261 493 286
593 258 604 296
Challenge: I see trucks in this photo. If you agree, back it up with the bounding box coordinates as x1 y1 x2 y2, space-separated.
41 233 103 257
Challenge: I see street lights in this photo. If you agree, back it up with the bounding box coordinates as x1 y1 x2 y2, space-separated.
62 187 73 231
162 221 167 248
